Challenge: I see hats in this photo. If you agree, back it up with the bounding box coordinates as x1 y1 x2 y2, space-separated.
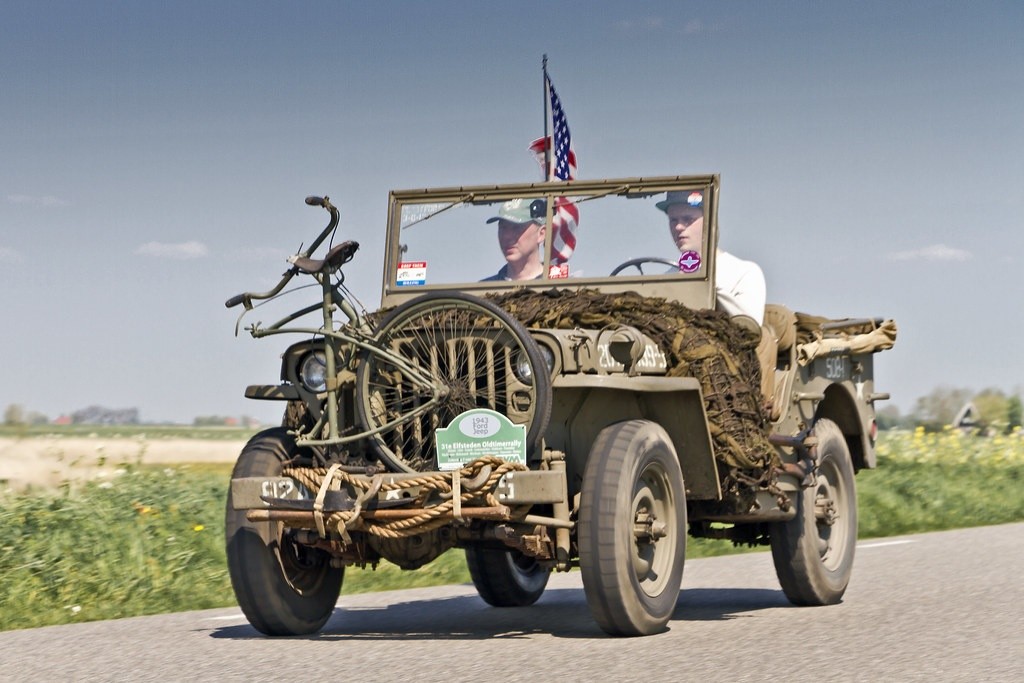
486 198 546 225
656 190 703 213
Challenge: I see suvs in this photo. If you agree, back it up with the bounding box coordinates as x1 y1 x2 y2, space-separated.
224 173 900 641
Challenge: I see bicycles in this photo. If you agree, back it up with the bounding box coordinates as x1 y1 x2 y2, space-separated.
225 197 552 483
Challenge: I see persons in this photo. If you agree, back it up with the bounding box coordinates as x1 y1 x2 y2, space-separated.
476 198 557 284
655 189 766 328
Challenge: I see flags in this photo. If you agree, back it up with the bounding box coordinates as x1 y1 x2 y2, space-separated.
528 73 578 267
